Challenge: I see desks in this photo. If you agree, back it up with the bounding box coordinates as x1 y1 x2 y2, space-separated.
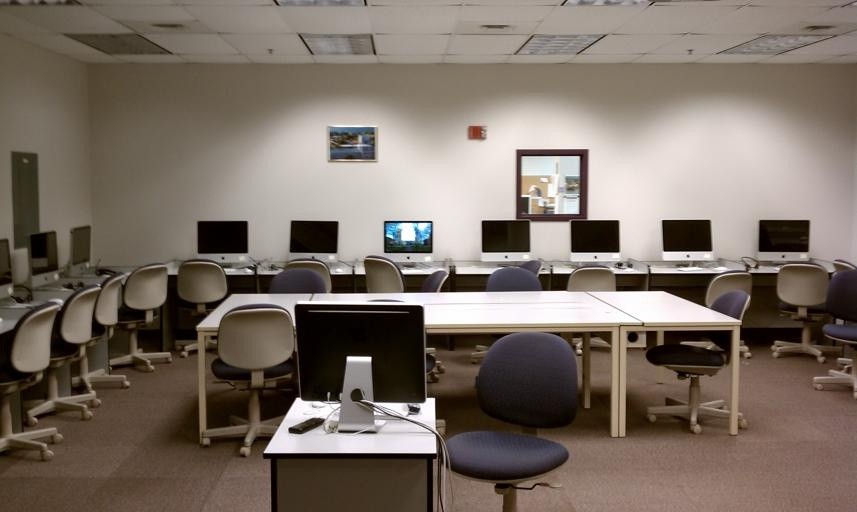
264 399 436 512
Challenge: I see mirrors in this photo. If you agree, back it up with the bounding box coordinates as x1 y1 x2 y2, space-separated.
515 148 589 219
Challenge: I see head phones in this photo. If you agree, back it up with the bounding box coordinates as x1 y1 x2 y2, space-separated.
10 285 32 302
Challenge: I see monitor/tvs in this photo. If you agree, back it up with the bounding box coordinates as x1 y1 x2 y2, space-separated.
288 219 339 262
569 218 622 265
383 219 433 263
294 301 428 433
660 218 715 267
481 219 533 263
0 238 14 302
71 224 91 273
758 217 812 265
195 219 250 264
29 231 61 289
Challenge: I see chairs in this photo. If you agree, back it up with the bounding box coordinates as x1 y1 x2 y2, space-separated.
441 333 578 512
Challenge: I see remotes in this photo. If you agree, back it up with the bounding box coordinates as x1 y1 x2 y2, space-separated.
290 417 327 433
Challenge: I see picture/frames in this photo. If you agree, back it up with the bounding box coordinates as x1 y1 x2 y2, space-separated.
326 125 378 162
